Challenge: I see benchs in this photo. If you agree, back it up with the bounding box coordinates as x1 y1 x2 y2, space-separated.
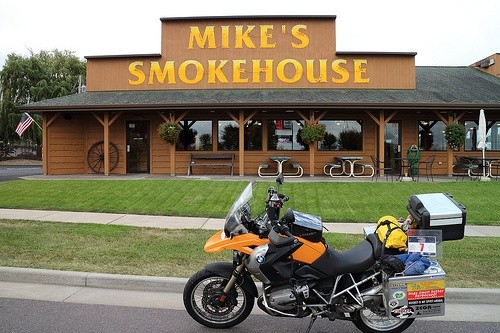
258 163 269 178
323 163 341 178
187 153 235 176
355 163 374 178
291 163 304 178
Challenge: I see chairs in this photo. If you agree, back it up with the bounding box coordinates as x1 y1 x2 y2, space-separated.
455 155 500 181
370 155 394 182
414 155 436 182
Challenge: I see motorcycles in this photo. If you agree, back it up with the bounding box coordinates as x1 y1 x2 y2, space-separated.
183 173 468 333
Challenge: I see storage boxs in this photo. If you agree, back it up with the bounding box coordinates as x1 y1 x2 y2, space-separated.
407 193 466 241
382 259 447 319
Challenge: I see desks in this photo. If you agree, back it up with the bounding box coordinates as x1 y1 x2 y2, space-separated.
394 158 420 182
270 156 291 178
335 155 363 178
464 156 500 178
